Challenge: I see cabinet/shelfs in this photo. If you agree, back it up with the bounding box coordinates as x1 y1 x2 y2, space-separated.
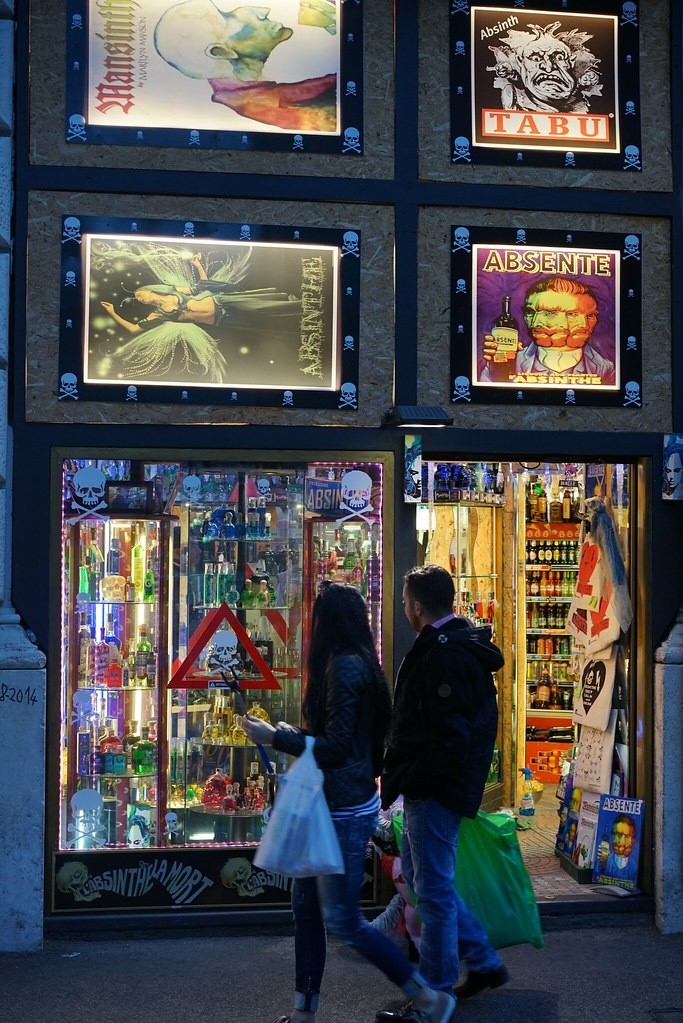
524 521 585 784
59 455 302 851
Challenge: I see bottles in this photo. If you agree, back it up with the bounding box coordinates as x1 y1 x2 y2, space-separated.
526 604 571 629
313 524 371 598
526 636 575 655
526 572 577 596
452 589 496 643
190 560 277 608
200 761 275 816
78 610 157 688
76 717 160 774
188 496 272 540
434 464 505 503
203 693 270 746
529 681 573 711
525 539 579 564
527 476 580 523
80 522 159 602
527 661 576 682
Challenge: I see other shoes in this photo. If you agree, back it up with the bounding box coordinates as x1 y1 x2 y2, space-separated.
375 988 458 1023
451 965 513 999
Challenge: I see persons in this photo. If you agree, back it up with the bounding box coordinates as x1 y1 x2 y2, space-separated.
242 584 455 1023
375 564 511 1023
111 487 131 508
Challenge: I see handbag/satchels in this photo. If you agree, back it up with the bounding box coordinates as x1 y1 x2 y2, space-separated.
252 736 347 878
390 805 545 957
